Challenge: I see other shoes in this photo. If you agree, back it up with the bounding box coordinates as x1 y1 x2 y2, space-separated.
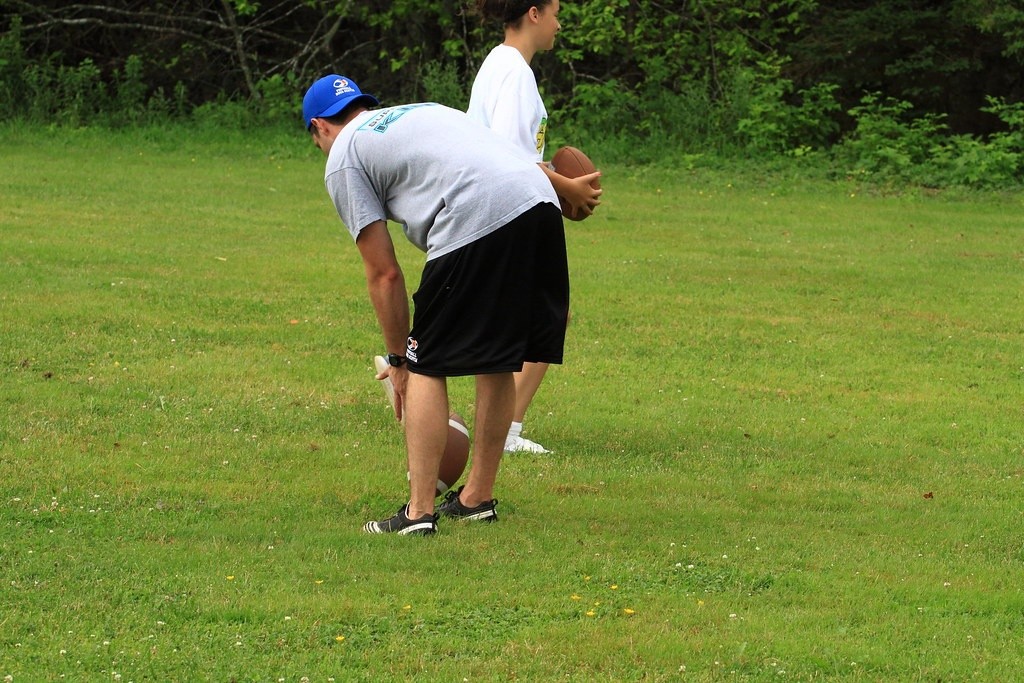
499 435 553 456
374 354 405 420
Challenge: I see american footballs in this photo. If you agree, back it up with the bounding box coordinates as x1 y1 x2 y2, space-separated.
403 409 471 502
545 145 602 222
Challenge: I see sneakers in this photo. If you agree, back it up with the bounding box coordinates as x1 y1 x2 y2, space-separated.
434 486 500 525
364 500 437 536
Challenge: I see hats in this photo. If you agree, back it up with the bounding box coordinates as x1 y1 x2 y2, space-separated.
299 74 379 131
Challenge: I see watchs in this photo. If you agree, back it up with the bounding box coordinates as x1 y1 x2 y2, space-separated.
387 353 406 367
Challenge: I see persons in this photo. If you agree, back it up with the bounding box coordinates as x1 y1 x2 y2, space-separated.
466 0 605 456
302 74 570 538
